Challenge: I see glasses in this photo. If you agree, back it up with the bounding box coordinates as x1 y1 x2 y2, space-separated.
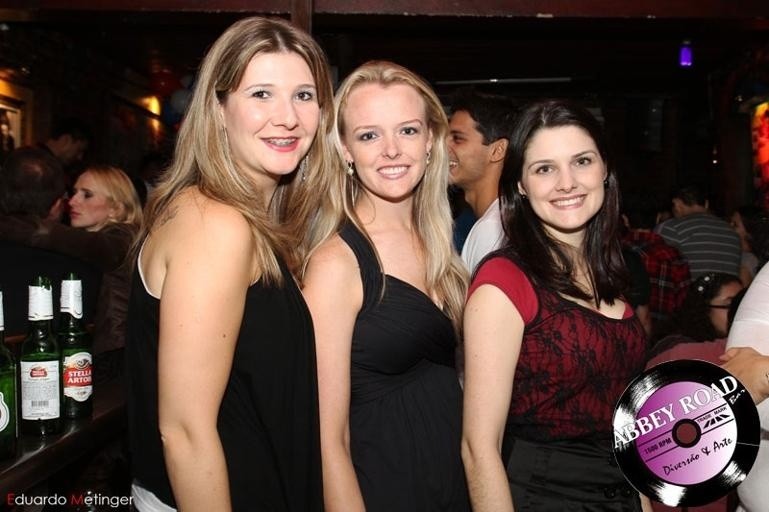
702 305 729 309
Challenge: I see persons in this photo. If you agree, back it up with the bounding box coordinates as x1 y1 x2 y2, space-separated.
0 16 768 511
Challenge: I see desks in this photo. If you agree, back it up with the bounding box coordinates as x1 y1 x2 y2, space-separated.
0 377 130 511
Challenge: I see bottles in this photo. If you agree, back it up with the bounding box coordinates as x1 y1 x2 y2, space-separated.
0 271 92 470
